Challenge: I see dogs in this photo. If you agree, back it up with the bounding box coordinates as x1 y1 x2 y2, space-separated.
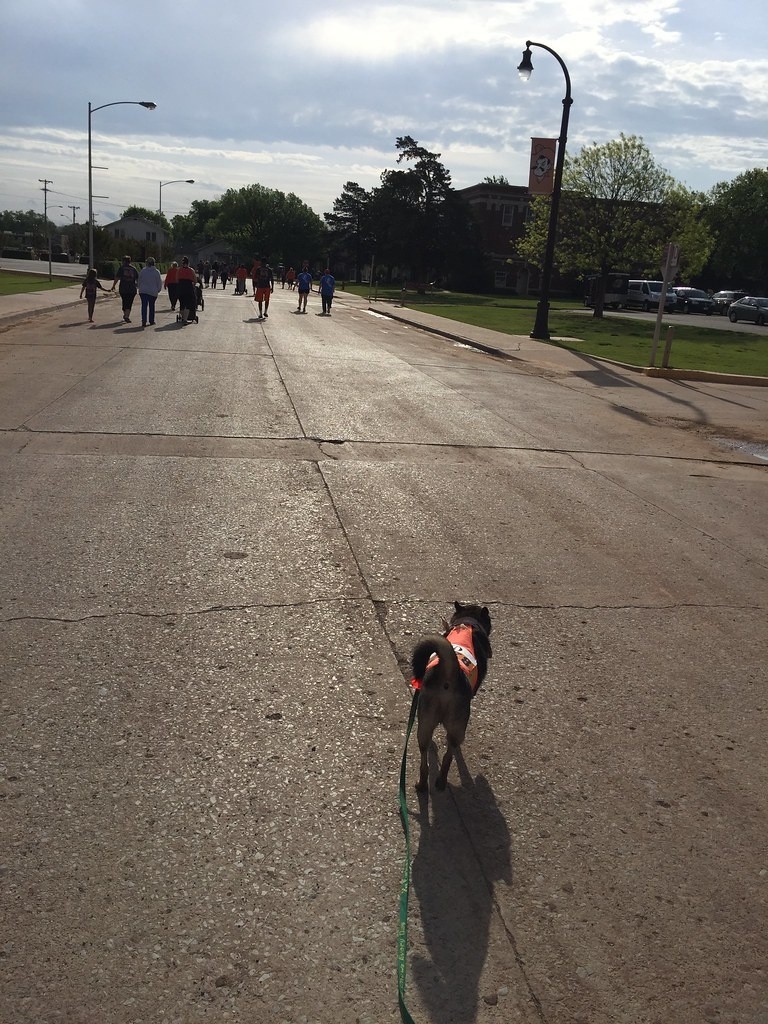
411 599 492 792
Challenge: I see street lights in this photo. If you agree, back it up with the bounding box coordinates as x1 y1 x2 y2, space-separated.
517 41 575 342
61 213 76 253
86 99 156 271
158 179 195 269
44 205 63 281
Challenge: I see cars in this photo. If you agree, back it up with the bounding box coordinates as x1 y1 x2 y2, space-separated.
711 290 750 317
727 297 768 326
671 287 715 316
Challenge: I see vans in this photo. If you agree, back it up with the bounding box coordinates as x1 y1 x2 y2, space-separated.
584 273 631 310
629 280 678 313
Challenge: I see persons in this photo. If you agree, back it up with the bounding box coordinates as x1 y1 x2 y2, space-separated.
138 257 162 327
197 259 235 289
236 264 246 294
112 255 139 323
254 259 274 317
317 269 335 314
176 256 196 325
79 269 111 322
164 262 180 310
266 263 313 290
251 261 261 295
293 267 312 313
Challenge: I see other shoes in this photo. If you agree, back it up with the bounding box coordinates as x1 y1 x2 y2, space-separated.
170 304 177 311
150 321 157 325
122 317 131 323
319 312 332 317
294 310 308 314
259 313 268 318
179 319 188 326
142 321 147 327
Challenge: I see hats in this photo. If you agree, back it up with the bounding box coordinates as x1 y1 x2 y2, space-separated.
324 269 329 274
302 265 309 272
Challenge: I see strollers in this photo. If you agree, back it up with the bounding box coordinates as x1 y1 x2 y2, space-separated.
175 285 199 323
193 278 205 311
234 279 248 295
